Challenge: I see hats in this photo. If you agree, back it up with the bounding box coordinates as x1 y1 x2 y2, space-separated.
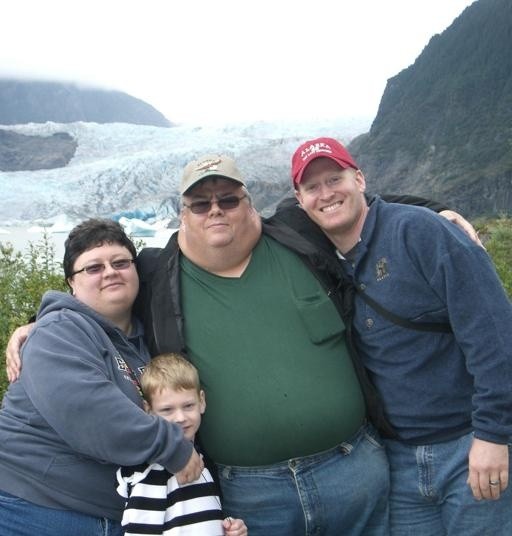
178 152 247 196
288 135 360 186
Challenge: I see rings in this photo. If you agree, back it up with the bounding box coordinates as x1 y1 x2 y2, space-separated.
200 465 204 472
489 480 499 486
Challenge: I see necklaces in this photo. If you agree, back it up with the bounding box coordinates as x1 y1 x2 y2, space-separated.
199 463 234 525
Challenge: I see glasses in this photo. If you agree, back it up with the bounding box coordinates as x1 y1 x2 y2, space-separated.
71 258 137 276
183 192 247 215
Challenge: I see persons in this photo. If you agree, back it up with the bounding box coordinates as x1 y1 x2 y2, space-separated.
0 218 206 536
1 153 486 536
114 351 249 536
289 137 511 535
